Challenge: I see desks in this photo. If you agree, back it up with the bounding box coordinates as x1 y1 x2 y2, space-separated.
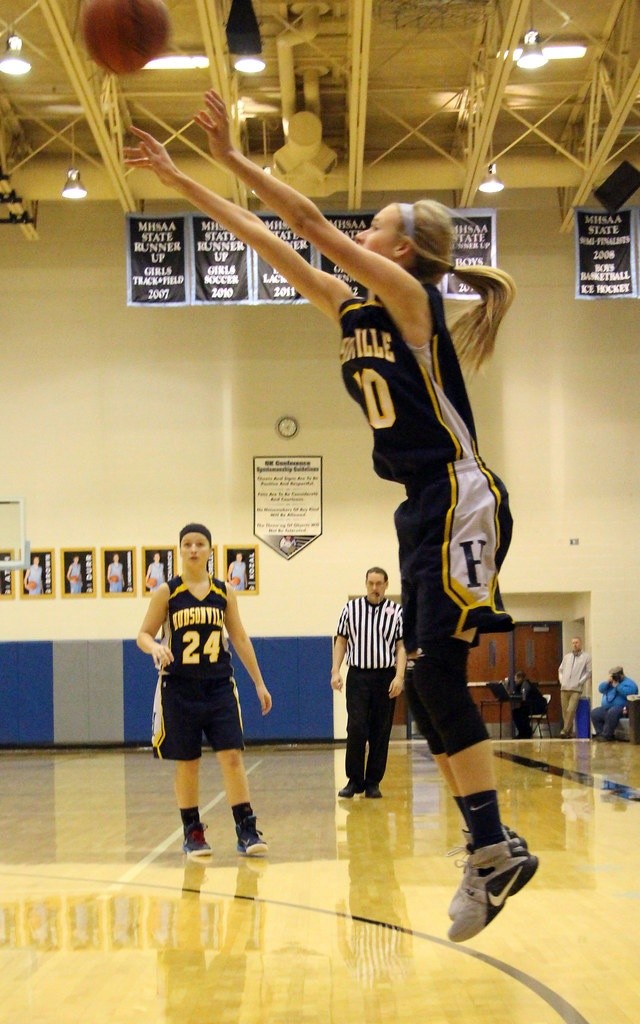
480 699 523 739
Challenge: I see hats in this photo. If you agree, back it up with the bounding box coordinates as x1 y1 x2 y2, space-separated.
608 667 623 677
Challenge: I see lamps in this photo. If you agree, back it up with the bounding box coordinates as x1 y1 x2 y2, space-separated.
0 10 32 74
478 136 504 193
61 125 87 199
516 0 549 69
497 40 587 59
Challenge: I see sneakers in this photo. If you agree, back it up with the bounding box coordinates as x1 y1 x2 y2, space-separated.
184 857 212 884
182 822 213 855
446 826 528 920
235 816 268 855
236 856 269 895
445 837 539 942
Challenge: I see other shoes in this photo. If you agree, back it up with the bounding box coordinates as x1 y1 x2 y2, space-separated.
561 729 571 738
340 800 360 812
364 780 381 798
597 734 614 742
512 731 532 739
338 779 365 797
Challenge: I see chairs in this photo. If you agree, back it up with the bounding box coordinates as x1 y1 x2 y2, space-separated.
528 694 552 739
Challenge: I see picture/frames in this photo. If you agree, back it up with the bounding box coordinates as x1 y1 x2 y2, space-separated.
101 546 138 597
60 547 98 598
222 544 260 595
19 548 56 599
142 545 177 597
182 545 217 577
0 548 16 600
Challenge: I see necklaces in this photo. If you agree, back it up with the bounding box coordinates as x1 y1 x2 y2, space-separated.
605 690 617 703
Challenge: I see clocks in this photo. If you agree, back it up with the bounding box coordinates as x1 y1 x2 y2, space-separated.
275 416 299 440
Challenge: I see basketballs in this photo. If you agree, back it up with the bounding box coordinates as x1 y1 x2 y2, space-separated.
234 577 240 585
147 578 157 586
79 0 170 76
28 581 36 589
112 576 119 582
70 575 78 582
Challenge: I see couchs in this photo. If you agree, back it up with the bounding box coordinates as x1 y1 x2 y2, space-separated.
613 694 640 745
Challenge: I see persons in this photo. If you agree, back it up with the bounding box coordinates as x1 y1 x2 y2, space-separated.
146 553 165 592
136 523 273 855
558 638 591 739
25 556 44 594
228 552 247 590
590 666 638 742
124 88 539 943
108 553 125 592
164 856 269 1024
334 799 414 1024
330 567 408 797
67 555 83 594
513 671 545 738
